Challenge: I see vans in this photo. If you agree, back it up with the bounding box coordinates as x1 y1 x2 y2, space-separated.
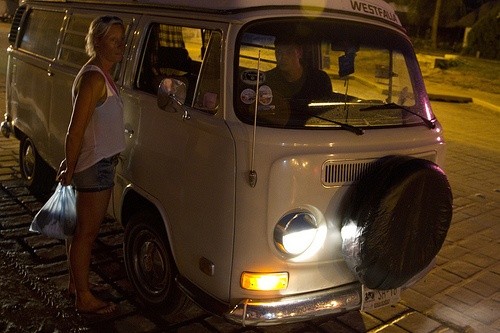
1 0 453 327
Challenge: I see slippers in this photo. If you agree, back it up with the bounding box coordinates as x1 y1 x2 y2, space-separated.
76 299 123 319
70 282 112 295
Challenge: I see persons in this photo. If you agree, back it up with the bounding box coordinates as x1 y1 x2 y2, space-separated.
256 31 332 128
57 16 127 312
